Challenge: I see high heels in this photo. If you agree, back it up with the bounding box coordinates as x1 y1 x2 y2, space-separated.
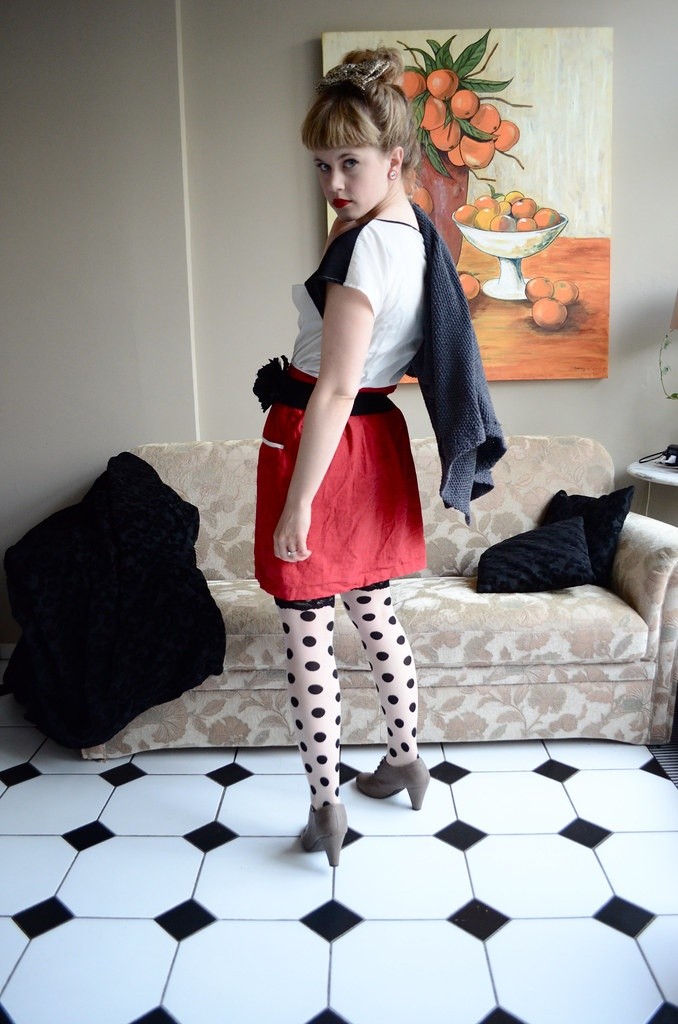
356 754 429 811
300 802 349 867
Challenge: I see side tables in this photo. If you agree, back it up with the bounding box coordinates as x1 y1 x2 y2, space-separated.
626 457 678 522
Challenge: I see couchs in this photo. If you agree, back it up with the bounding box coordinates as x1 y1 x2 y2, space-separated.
3 439 678 759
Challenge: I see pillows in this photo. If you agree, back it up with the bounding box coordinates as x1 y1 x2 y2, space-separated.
478 518 595 593
537 485 634 586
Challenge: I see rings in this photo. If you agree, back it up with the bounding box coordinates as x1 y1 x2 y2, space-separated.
287 551 297 556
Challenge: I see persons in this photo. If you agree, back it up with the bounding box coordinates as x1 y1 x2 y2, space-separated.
254 48 431 867
